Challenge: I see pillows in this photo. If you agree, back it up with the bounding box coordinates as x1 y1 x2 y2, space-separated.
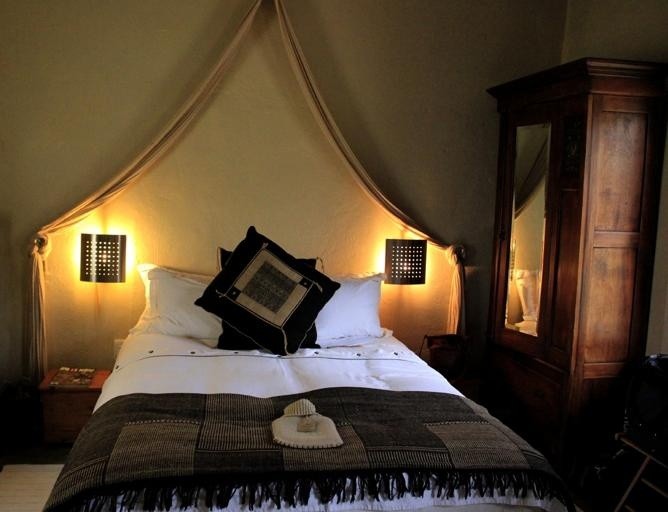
515 270 541 322
141 226 386 354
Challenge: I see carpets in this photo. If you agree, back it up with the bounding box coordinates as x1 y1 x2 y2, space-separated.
1 464 65 511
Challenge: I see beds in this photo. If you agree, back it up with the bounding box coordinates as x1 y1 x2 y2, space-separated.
43 264 583 511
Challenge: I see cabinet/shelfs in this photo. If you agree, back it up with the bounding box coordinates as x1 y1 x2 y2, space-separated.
485 58 667 437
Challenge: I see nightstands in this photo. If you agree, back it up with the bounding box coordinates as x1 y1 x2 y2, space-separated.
42 389 101 446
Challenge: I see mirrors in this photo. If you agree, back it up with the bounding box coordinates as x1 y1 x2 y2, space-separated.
502 119 554 341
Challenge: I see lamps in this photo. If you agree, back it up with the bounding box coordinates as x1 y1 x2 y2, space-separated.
385 240 426 284
80 232 128 282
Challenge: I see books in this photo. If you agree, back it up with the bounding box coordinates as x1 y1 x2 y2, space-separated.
48 366 96 388
